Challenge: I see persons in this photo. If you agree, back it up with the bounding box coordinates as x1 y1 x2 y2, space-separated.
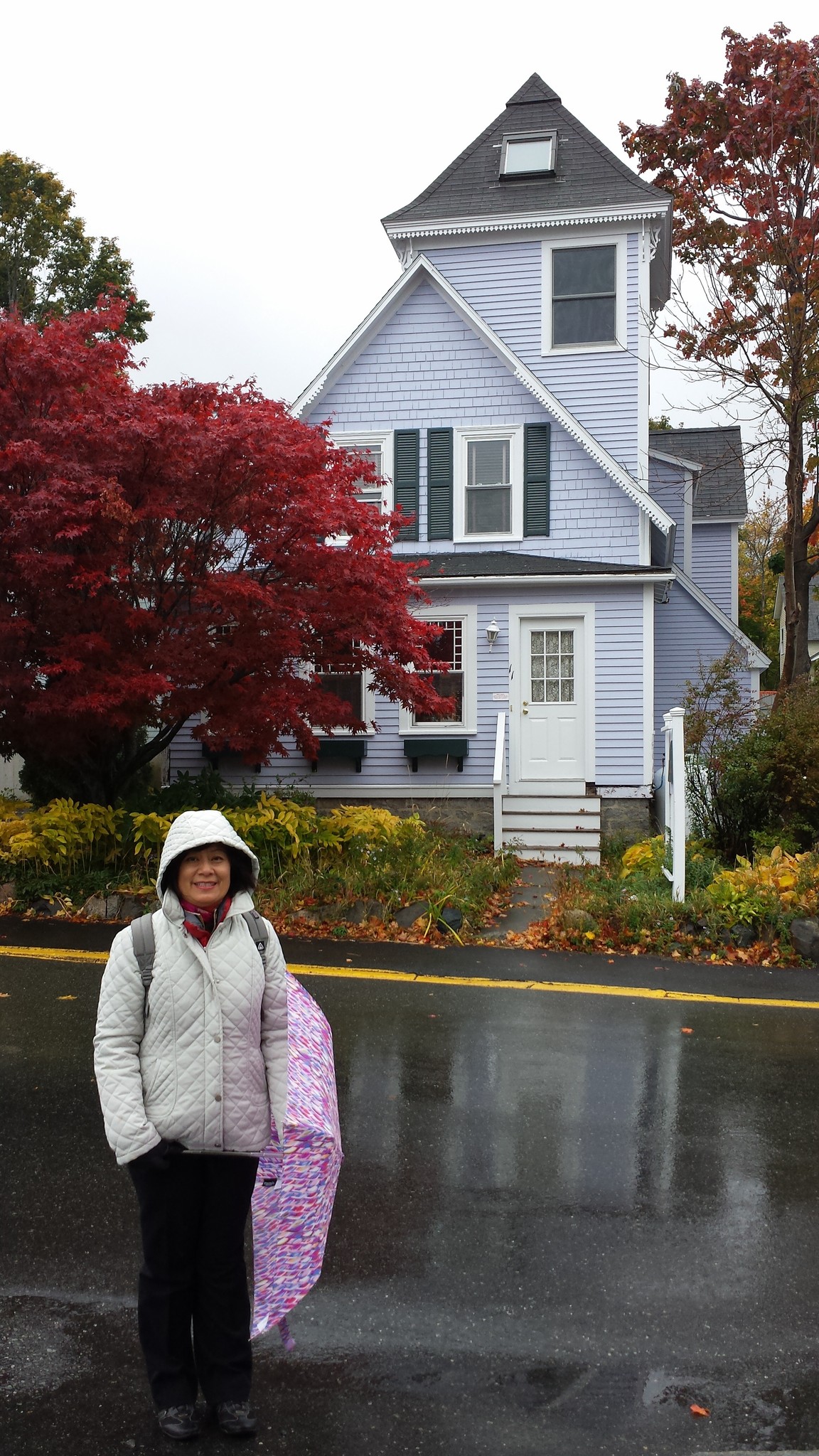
93 807 290 1441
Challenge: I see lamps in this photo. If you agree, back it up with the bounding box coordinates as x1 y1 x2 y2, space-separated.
484 616 501 653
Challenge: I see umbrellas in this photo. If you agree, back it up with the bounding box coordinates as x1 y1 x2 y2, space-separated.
148 970 347 1356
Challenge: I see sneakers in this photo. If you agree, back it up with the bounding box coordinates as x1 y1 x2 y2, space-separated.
211 1397 256 1436
157 1404 202 1440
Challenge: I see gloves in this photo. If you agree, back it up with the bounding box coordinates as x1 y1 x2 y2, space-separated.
128 1140 182 1191
